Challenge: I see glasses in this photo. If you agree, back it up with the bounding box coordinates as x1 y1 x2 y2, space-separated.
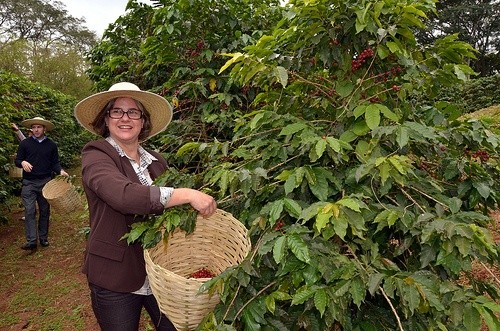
106 107 146 120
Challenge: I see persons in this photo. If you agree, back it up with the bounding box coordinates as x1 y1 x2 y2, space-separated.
87 84 218 331
10 122 34 141
14 117 70 250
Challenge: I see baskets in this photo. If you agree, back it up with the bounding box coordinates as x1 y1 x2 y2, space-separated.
42 177 81 215
9 154 22 178
144 207 251 331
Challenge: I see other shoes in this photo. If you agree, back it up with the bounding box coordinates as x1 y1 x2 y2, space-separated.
39 241 49 247
21 243 37 250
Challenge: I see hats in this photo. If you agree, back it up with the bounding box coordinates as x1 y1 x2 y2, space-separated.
21 117 54 131
74 82 173 143
